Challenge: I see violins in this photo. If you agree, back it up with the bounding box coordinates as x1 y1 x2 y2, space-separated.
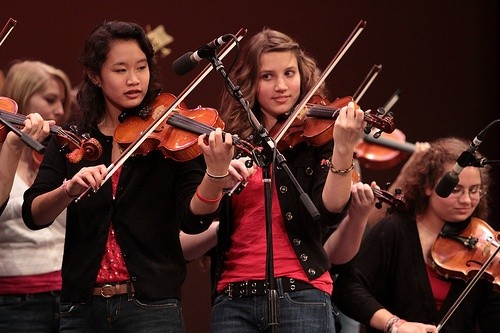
353 125 415 169
114 92 276 169
0 95 102 164
428 217 500 292
266 93 395 149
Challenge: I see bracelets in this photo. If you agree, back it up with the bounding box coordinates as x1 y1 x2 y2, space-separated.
386 316 406 333
205 169 231 179
195 186 223 204
61 180 77 199
327 155 356 174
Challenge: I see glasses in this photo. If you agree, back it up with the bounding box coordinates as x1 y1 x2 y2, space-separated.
449 186 487 200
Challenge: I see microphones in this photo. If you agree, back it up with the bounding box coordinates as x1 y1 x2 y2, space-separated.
172 34 232 76
434 130 487 197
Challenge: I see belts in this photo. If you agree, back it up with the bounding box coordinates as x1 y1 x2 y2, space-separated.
91 283 135 297
223 276 315 300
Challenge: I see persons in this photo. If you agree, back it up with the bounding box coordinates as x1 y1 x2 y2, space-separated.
22 22 236 332
0 111 51 218
333 138 500 333
1 61 81 333
228 31 365 333
323 180 381 265
